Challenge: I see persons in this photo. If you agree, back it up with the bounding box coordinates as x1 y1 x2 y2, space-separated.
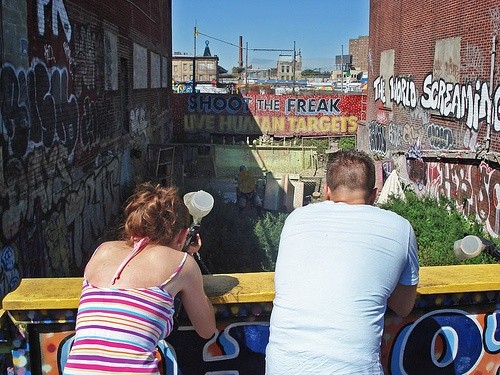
61 177 216 375
171 77 271 97
264 151 422 374
